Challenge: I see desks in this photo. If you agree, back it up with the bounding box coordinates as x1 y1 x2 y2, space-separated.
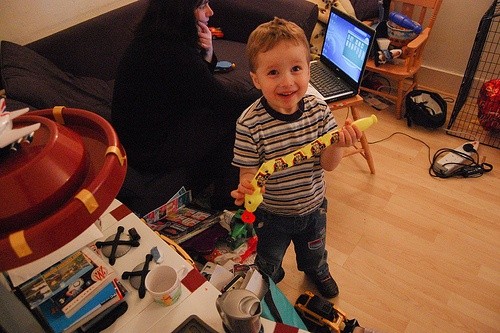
326 93 375 175
0 199 312 333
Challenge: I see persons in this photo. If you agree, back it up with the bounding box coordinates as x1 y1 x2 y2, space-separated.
230 17 363 300
111 0 251 210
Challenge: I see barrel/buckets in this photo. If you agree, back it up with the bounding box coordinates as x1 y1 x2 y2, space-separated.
386 19 421 60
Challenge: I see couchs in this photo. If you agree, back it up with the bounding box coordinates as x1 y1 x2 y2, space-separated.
0 0 318 219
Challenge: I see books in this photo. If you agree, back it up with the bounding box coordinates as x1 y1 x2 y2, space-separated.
7 246 129 333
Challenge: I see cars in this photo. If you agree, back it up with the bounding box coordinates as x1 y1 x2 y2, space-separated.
294 290 348 333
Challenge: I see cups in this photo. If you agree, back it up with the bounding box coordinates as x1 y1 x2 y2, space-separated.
144 265 182 306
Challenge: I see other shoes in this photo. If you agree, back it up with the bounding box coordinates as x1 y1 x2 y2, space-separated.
307 264 340 298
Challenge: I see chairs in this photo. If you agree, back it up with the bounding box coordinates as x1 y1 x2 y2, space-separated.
359 0 443 120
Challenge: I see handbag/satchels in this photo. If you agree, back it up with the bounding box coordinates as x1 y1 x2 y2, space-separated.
404 89 449 128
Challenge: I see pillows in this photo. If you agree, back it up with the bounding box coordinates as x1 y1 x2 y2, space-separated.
0 40 116 125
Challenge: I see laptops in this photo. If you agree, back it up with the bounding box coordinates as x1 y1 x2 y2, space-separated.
306 6 376 104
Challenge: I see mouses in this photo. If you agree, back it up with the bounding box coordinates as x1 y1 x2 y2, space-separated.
214 60 236 73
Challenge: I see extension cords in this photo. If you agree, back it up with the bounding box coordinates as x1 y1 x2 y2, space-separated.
434 140 479 176
